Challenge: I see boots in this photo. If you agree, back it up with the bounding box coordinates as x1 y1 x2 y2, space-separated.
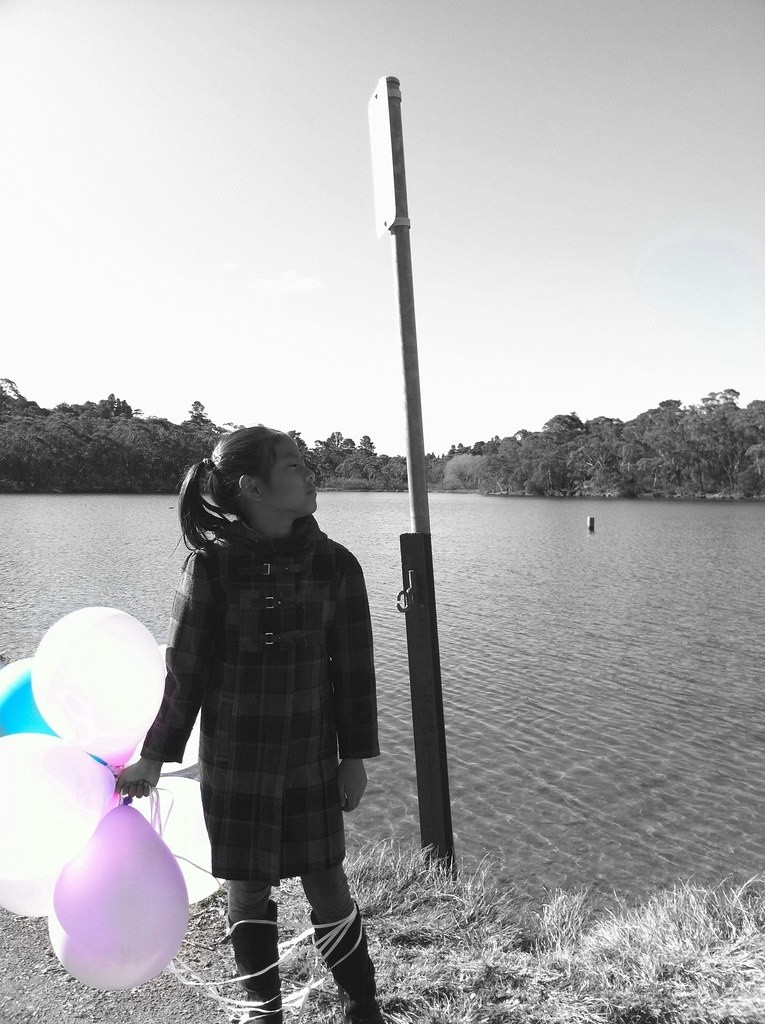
228 899 282 1024
311 898 387 1024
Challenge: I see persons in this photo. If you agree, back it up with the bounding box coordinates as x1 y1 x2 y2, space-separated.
113 425 385 1024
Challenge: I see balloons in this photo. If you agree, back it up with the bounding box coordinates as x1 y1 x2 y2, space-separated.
0 607 227 993
